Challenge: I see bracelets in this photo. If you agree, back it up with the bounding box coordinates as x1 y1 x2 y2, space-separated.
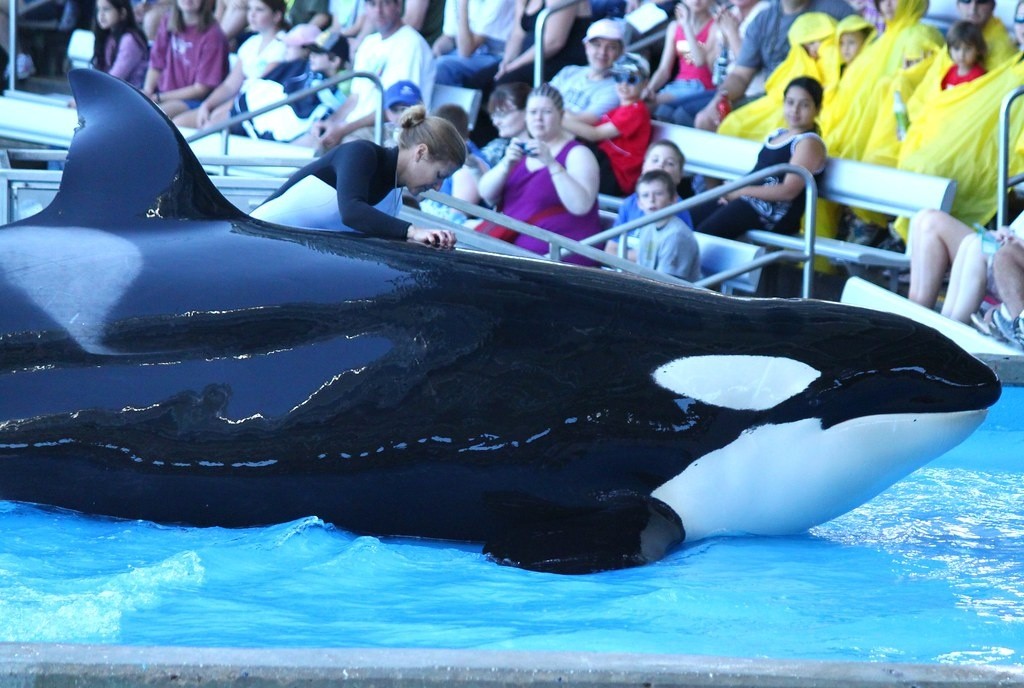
156 91 161 104
550 165 566 178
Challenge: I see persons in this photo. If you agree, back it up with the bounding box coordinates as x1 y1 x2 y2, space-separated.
560 52 653 198
477 83 601 272
248 102 468 248
601 138 693 272
382 80 532 230
633 169 706 286
547 17 628 141
637 1 1023 289
0 0 638 152
909 206 1024 354
694 76 827 242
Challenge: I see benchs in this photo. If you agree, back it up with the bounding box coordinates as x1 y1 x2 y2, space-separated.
430 82 956 298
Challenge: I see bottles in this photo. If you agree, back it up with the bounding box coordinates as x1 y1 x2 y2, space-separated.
712 47 727 86
895 91 910 139
714 90 731 122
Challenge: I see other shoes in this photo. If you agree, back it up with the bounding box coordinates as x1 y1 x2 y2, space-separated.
5 54 37 81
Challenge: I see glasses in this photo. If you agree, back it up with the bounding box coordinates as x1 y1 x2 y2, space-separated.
491 103 517 120
611 75 642 82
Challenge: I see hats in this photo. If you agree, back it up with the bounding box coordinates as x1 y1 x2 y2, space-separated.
383 80 423 110
301 33 350 62
583 18 632 51
607 52 652 78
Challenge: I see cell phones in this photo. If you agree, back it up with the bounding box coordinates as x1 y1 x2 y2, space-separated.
515 142 537 156
